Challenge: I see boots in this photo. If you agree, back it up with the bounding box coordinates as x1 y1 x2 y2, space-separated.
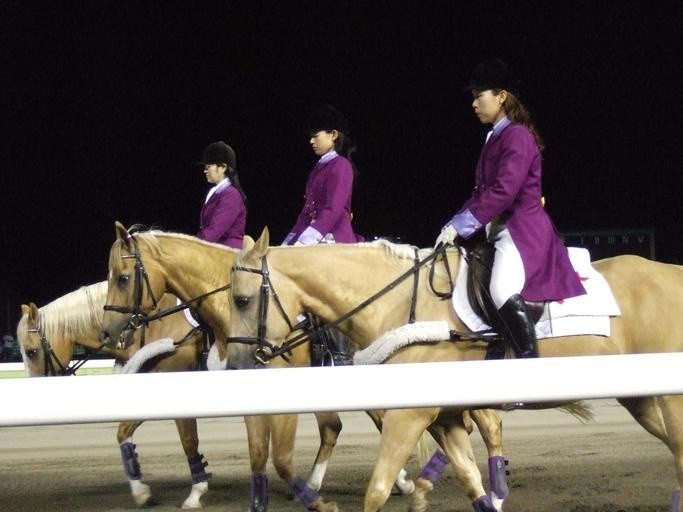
495 294 539 411
318 328 354 365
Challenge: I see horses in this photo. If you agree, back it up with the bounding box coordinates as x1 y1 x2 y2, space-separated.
97 221 422 512
223 224 683 511
15 279 216 511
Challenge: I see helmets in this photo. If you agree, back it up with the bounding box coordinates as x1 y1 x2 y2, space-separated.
193 141 236 168
304 105 350 130
462 58 523 91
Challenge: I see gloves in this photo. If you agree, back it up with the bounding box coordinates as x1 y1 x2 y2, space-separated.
433 225 458 248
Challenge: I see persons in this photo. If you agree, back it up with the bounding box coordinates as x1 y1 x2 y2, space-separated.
277 103 363 367
192 142 248 249
433 54 587 359
